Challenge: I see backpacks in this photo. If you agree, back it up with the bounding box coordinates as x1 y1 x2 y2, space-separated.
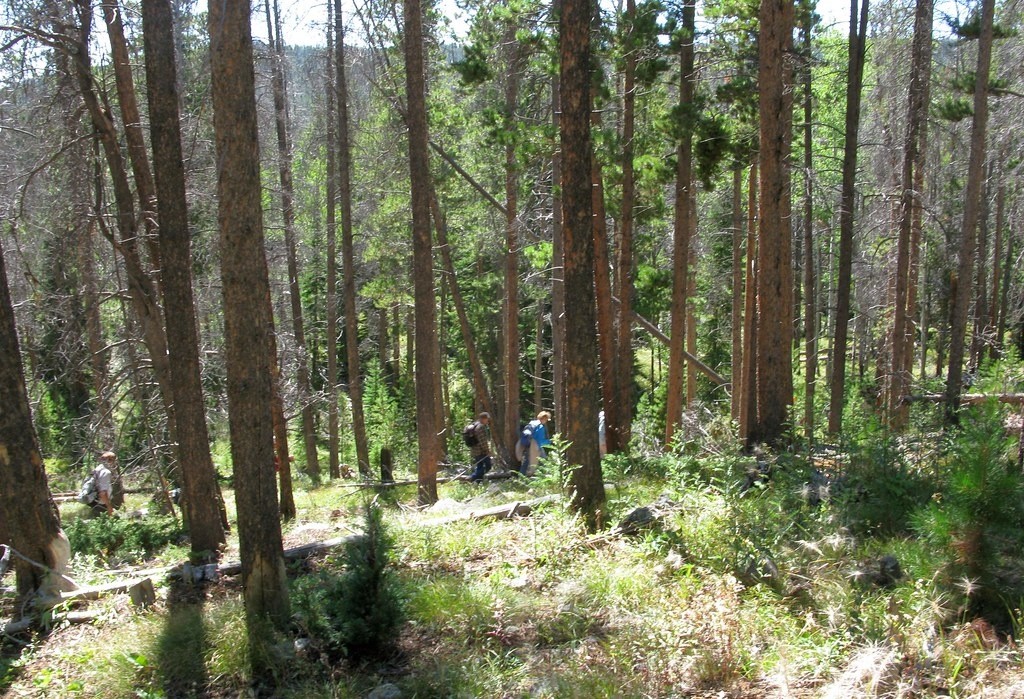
462 424 479 448
76 469 104 505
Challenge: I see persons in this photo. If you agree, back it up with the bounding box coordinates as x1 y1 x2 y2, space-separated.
460 412 491 484
87 451 116 517
520 410 551 477
273 435 295 471
599 411 608 454
